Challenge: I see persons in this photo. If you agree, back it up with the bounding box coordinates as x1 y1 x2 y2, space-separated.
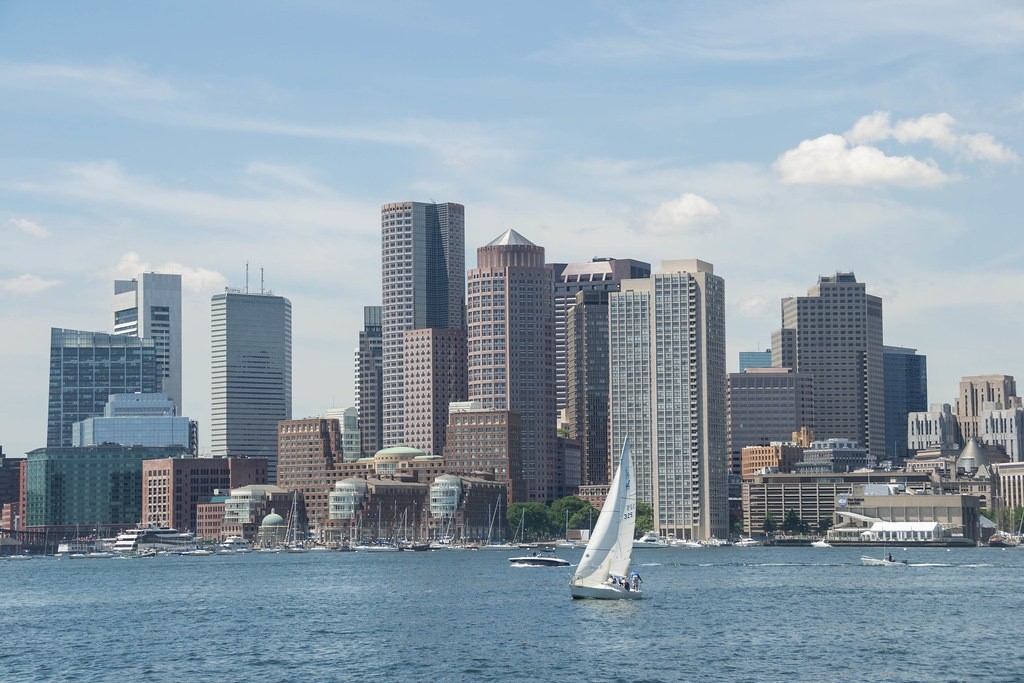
610 575 630 592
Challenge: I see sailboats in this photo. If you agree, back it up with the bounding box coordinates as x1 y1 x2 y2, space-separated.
988 502 1023 548
41 522 109 558
279 488 592 552
568 431 646 600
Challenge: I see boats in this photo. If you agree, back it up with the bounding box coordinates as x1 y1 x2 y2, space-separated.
508 550 571 567
110 522 279 557
632 530 759 549
859 540 906 566
810 537 833 548
